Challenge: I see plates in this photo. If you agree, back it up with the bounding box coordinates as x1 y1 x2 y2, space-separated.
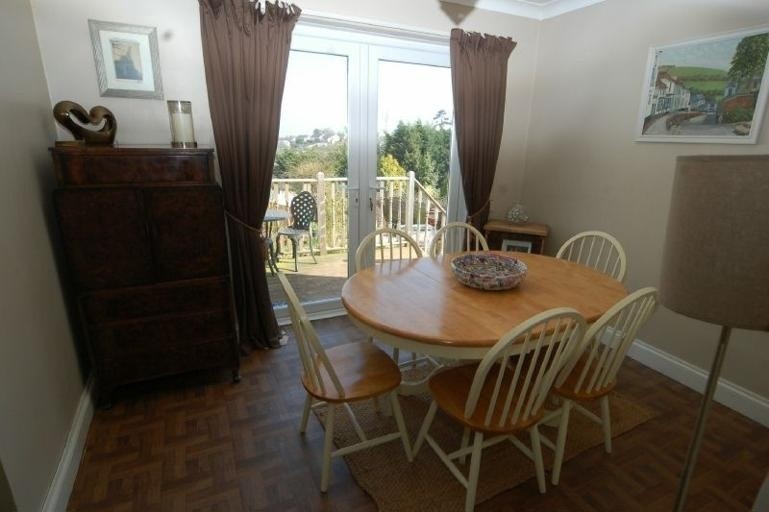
450 253 528 290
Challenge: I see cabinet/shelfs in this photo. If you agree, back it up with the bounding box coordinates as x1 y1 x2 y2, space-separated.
45 146 243 411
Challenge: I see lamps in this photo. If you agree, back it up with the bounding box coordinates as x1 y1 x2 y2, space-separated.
661 153 769 512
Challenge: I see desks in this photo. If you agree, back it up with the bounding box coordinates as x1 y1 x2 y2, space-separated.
482 219 548 255
262 210 287 253
343 248 628 466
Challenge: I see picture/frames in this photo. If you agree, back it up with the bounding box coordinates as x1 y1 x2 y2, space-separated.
632 21 769 145
88 20 164 102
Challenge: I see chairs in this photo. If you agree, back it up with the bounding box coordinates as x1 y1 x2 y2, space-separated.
276 272 415 493
273 191 319 271
256 236 279 276
501 287 661 487
410 306 588 512
513 229 628 407
353 228 450 410
428 223 512 388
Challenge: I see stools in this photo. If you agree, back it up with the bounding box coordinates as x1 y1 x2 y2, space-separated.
500 238 532 253
